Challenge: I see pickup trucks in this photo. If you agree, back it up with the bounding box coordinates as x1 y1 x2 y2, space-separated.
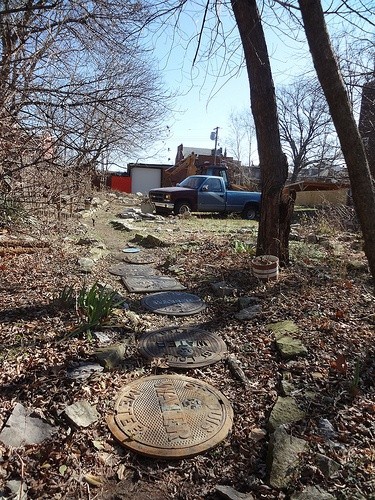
147 174 261 221
